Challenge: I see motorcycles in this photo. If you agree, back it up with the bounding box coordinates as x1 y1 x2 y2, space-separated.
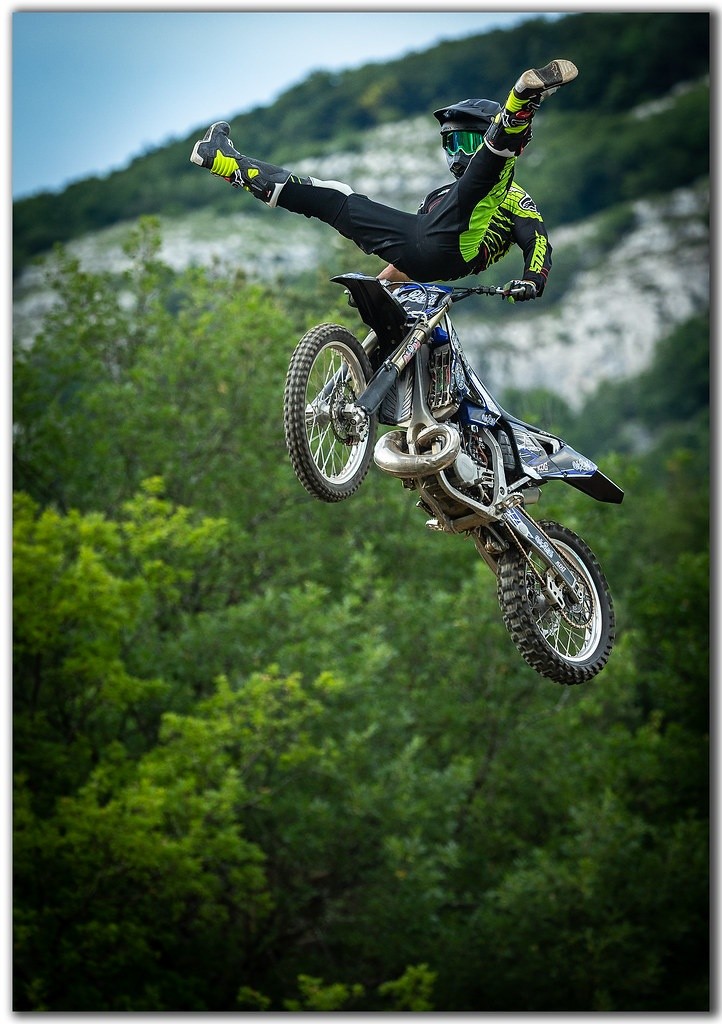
284 273 627 685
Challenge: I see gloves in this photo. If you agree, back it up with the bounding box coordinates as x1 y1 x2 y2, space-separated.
501 270 543 303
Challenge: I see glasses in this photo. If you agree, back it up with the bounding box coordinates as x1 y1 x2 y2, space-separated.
439 130 483 154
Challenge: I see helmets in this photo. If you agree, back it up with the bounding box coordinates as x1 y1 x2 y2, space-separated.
432 97 504 177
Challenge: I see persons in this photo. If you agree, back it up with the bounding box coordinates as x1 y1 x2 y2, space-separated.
189 60 579 308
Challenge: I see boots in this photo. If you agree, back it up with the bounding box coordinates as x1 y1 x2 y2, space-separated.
484 57 576 157
189 120 292 210
